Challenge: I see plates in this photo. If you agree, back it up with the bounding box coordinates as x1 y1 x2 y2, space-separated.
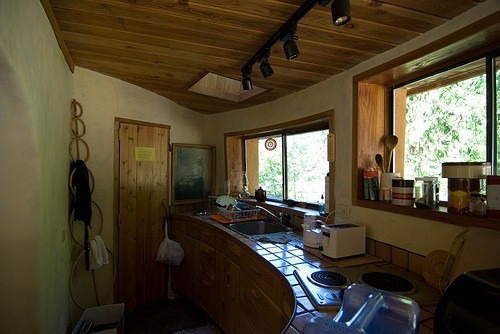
216 195 238 206
423 250 456 290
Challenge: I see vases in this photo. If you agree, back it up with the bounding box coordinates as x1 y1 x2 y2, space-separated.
238 171 250 199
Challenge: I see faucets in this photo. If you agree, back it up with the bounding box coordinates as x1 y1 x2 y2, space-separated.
255 205 284 224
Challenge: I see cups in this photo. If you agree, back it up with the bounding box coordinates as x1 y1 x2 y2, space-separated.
379 172 401 201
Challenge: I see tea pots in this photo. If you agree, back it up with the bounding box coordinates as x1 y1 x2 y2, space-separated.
255 187 267 203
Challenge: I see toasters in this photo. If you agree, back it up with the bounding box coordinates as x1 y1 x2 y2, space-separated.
321 221 365 259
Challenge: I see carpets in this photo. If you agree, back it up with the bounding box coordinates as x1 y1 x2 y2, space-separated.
173 322 221 334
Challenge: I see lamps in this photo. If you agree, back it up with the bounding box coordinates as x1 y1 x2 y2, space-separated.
331 0 351 26
256 48 275 79
241 65 252 90
279 24 301 60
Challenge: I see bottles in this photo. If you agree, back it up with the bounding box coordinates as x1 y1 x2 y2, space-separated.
415 177 437 209
441 163 492 180
391 178 414 206
319 194 325 214
324 172 329 214
224 179 230 194
382 187 391 202
447 178 480 214
486 175 500 217
469 194 486 217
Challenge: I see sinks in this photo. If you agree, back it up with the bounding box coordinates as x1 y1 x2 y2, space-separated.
229 221 292 239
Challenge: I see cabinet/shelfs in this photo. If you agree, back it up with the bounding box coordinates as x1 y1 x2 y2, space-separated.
172 217 295 334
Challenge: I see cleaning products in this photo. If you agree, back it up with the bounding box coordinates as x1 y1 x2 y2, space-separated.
318 195 325 215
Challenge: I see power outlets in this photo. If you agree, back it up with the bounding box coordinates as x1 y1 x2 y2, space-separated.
335 203 350 219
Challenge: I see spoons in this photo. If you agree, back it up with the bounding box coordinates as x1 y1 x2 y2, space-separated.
374 154 384 173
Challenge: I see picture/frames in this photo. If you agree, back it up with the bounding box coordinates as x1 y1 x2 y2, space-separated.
171 143 217 205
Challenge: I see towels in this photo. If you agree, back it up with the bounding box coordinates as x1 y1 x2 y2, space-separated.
88 235 110 270
73 159 93 230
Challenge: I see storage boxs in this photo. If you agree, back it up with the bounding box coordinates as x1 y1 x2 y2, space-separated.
72 303 126 334
